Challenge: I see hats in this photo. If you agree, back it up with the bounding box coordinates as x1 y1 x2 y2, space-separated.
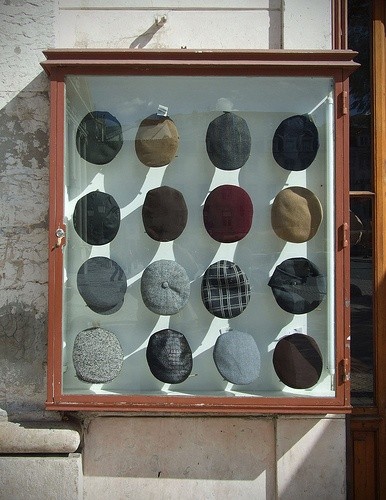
140 260 190 315
75 111 123 165
77 257 127 316
146 329 193 384
136 113 177 167
72 327 123 383
272 115 320 171
268 258 327 314
203 186 253 243
272 333 324 389
142 186 188 242
271 186 324 243
214 330 259 384
73 191 121 246
206 113 252 170
201 260 251 319
